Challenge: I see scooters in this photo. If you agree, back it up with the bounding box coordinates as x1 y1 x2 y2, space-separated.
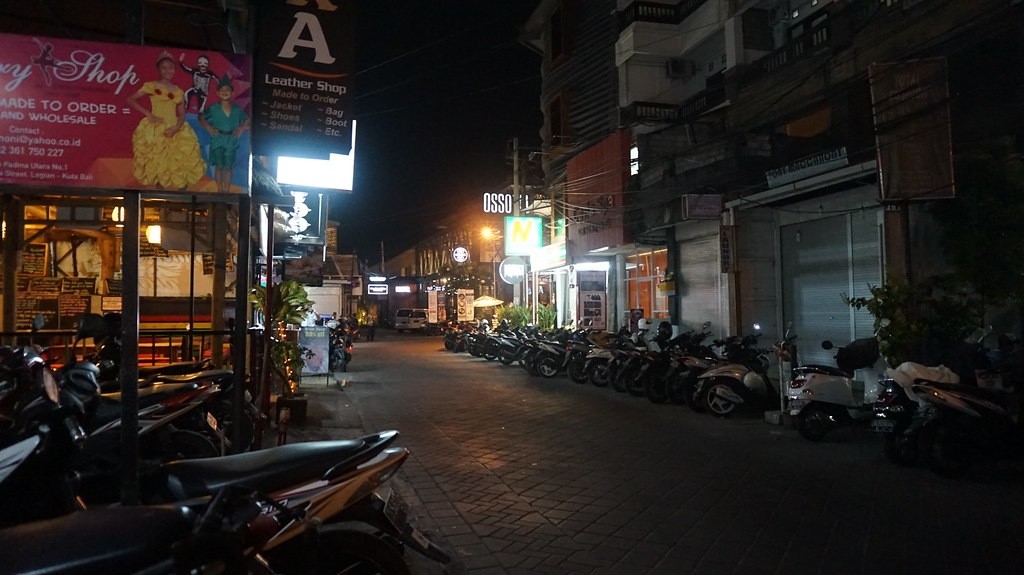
440 316 776 417
870 325 1024 466
786 319 893 441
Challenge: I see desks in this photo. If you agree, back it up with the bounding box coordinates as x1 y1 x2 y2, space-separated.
48 342 181 363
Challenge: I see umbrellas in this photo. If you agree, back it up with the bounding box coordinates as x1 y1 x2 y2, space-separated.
473 295 504 307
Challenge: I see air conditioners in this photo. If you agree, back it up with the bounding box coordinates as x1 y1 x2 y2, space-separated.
768 4 789 27
667 58 692 80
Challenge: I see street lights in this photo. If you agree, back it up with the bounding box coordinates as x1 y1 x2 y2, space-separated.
482 228 495 296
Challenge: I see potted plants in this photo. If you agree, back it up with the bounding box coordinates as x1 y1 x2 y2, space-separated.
250 279 317 429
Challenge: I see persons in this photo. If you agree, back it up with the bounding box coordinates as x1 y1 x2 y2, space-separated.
199 81 250 193
127 56 207 190
351 313 358 327
366 308 377 341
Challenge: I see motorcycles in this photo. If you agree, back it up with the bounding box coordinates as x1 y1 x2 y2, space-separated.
0 312 451 575
326 313 353 371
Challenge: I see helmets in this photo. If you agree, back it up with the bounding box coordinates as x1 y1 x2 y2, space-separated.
894 362 930 388
658 322 673 339
638 318 650 330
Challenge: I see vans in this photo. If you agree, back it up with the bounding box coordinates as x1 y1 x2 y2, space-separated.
408 309 429 333
394 307 410 333
